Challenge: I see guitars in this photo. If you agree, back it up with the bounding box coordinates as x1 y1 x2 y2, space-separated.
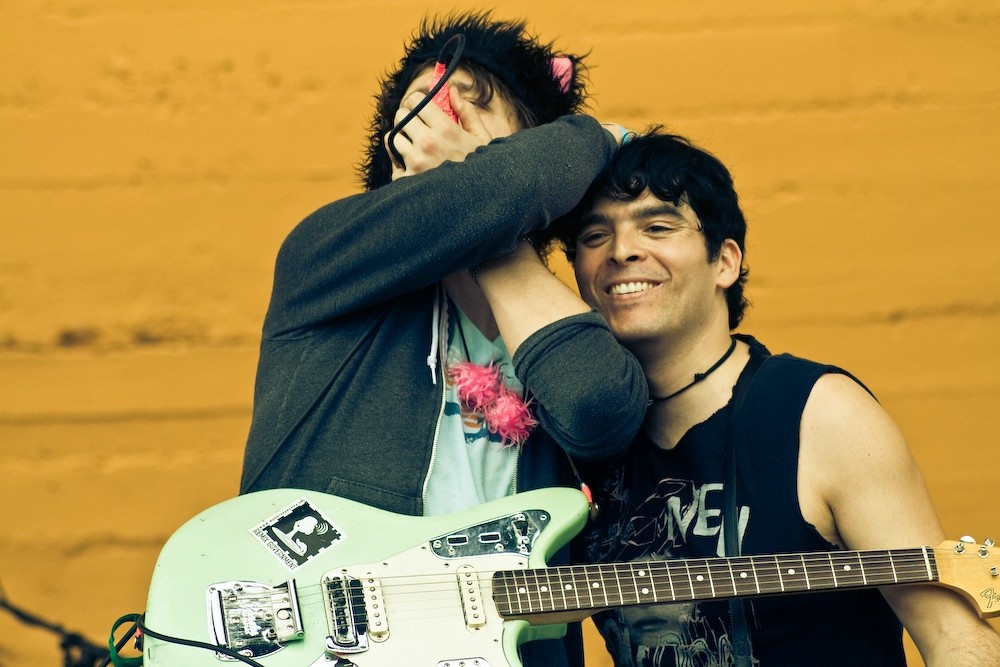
139 486 999 667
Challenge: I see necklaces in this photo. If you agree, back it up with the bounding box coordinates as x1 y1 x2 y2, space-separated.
649 337 737 402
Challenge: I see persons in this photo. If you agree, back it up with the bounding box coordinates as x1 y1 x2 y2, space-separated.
238 7 654 666
554 122 1000 666
